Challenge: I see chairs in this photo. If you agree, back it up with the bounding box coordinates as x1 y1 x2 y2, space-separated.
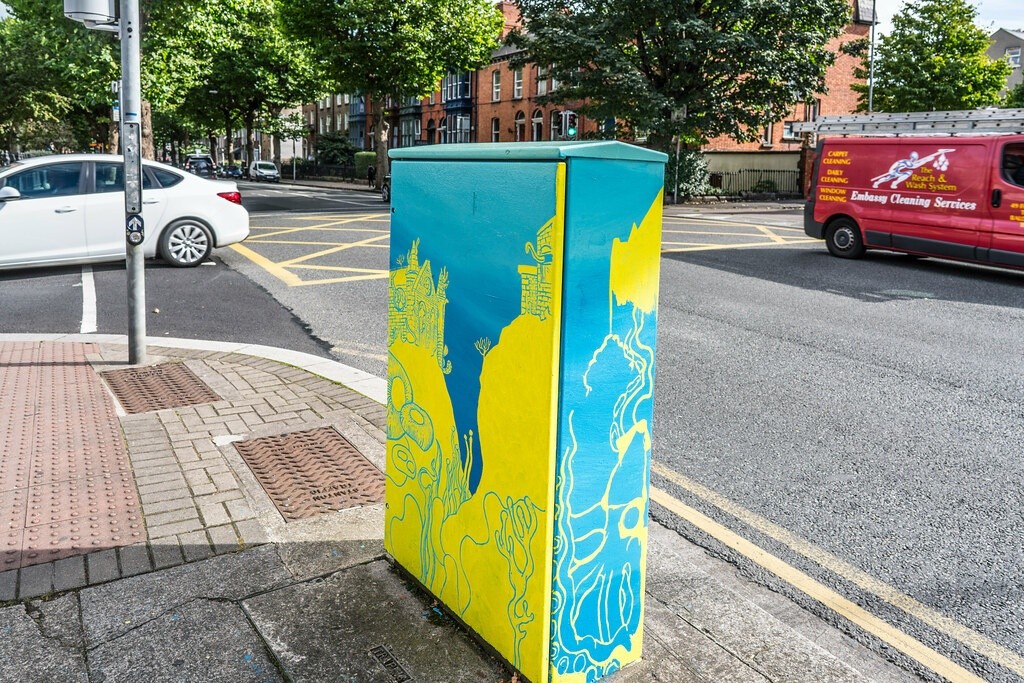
49 172 76 191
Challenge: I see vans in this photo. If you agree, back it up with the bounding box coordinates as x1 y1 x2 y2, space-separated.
803 134 1023 277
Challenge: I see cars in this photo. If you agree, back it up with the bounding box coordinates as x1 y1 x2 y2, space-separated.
184 154 244 180
0 150 251 273
249 160 281 183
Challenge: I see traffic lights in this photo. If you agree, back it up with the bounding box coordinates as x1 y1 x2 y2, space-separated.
567 113 576 136
